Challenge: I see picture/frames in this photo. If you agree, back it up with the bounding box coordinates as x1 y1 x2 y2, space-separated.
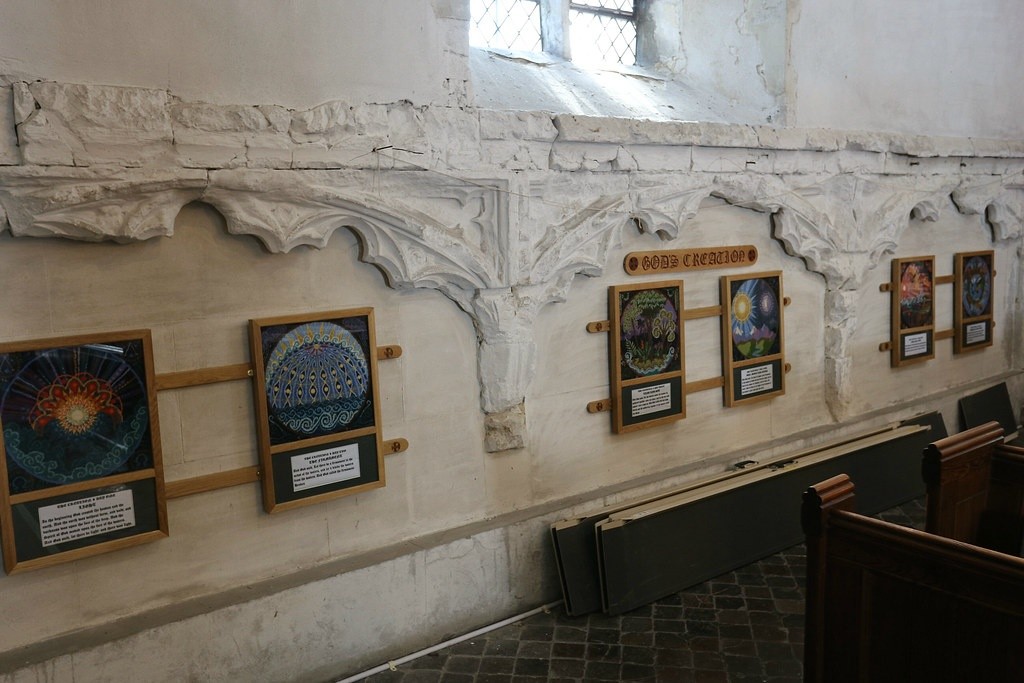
892 255 936 368
248 306 386 515
609 280 686 435
955 250 994 354
0 329 170 576
721 270 786 408
